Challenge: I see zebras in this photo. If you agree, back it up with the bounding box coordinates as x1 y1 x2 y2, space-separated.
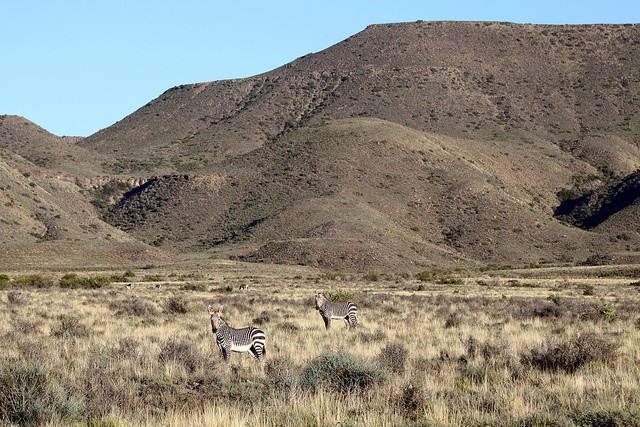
207 304 266 363
238 284 248 290
314 293 358 329
